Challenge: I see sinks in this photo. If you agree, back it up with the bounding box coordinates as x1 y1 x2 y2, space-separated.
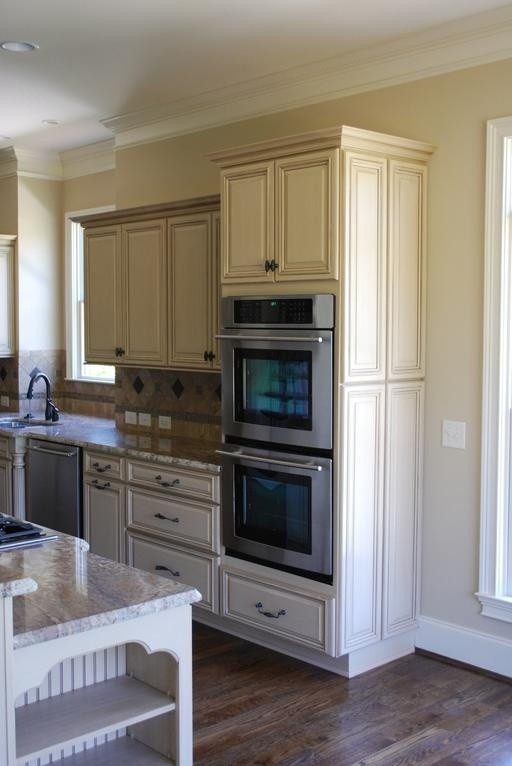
0 416 56 432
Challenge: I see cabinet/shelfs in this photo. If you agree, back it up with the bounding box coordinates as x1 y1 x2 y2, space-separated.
167 195 222 374
5 604 192 766
202 123 427 297
220 557 349 677
83 449 124 565
124 456 220 631
83 201 167 370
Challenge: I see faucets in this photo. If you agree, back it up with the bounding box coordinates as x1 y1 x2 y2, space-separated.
26 373 60 422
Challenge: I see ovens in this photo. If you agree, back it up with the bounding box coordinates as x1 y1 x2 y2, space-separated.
215 293 336 451
216 441 333 586
